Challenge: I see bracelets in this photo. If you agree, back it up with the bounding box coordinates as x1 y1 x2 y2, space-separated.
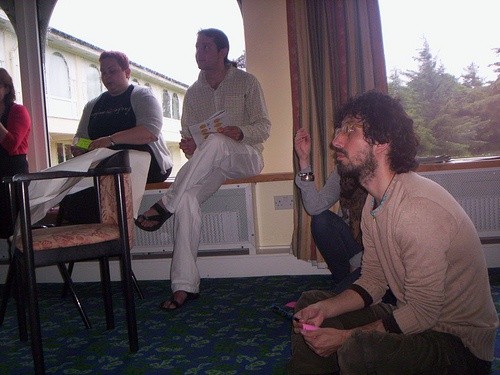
299 172 315 181
108 136 115 146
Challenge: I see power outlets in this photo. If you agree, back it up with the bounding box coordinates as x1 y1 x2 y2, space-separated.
273 195 293 210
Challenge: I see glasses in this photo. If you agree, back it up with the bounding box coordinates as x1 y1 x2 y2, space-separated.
334 122 362 138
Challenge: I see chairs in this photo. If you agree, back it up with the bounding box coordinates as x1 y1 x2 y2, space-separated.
2 150 139 375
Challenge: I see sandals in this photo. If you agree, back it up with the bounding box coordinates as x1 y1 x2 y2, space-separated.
135 203 172 232
160 292 200 311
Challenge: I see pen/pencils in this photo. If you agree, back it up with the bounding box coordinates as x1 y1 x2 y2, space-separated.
272 304 315 327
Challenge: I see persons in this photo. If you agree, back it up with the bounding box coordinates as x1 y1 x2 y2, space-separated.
135 29 272 311
286 91 500 375
57 51 171 225
0 68 33 240
295 127 367 285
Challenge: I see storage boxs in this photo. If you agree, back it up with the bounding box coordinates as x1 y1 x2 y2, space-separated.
73 137 93 150
